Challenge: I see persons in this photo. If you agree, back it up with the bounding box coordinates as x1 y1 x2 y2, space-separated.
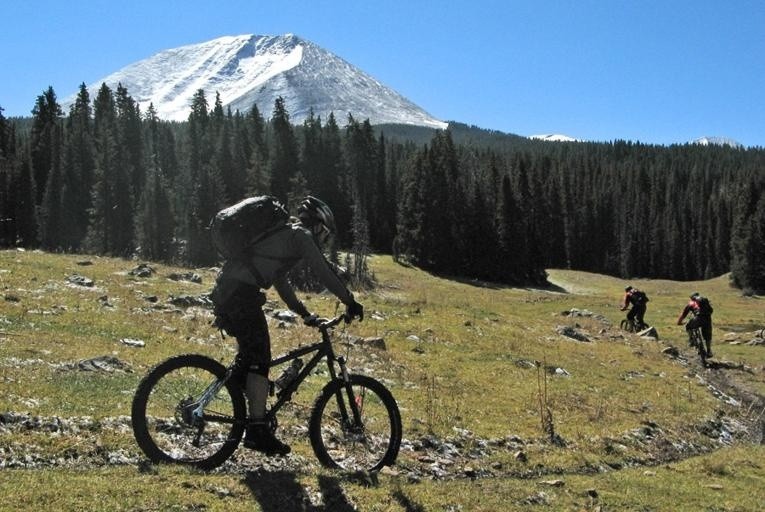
619 285 646 333
213 191 363 460
676 291 714 359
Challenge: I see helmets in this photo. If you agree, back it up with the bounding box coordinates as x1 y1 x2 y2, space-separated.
297 195 337 232
625 286 632 291
690 292 698 298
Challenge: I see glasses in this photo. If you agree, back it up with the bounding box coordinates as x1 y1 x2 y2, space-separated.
322 224 331 244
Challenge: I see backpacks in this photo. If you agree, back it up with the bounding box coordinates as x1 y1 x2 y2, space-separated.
210 195 303 260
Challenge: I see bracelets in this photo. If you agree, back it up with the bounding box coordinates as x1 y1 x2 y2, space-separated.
338 289 354 304
290 302 305 316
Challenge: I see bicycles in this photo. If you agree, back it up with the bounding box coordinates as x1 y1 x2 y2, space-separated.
129 302 403 483
616 307 649 334
678 317 708 365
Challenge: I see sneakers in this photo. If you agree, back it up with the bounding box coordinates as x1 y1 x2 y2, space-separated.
244 423 291 454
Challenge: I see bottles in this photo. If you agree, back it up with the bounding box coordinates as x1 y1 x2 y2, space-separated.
685 316 696 321
273 357 304 392
